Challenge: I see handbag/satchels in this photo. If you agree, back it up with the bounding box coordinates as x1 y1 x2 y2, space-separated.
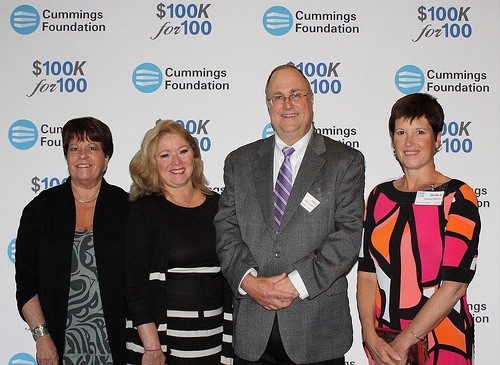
374 328 430 365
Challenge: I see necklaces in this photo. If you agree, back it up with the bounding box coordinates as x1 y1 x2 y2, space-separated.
71 188 100 204
163 186 194 206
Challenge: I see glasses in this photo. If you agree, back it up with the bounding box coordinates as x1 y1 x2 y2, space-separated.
267 93 310 105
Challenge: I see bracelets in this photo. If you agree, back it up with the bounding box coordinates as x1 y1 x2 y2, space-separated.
30 323 51 341
144 347 161 352
408 321 426 341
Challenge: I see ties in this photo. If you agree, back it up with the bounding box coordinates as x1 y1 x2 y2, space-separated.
273 147 296 234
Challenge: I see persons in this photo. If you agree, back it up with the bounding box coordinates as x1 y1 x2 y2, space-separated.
212 64 365 365
15 116 130 365
355 92 482 365
121 120 234 365
